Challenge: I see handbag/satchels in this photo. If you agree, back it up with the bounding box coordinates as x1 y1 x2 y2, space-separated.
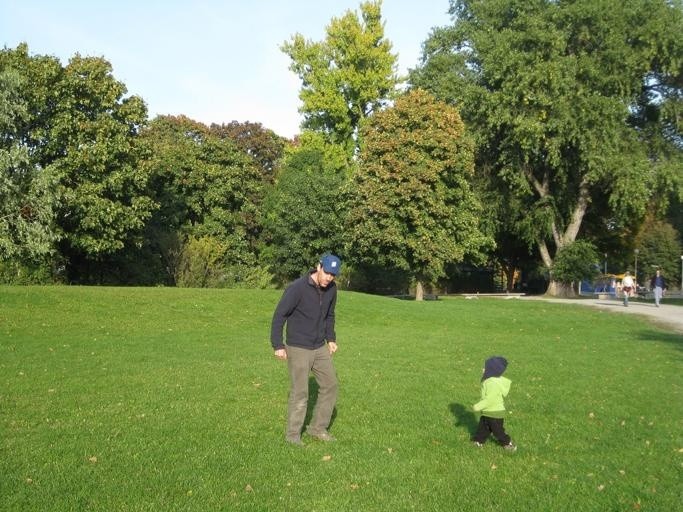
624 287 631 292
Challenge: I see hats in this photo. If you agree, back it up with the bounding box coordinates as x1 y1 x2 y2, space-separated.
481 357 508 383
320 255 341 275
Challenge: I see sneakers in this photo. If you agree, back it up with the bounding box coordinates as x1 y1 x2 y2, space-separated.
286 432 306 446
307 425 336 442
470 440 483 447
505 442 517 452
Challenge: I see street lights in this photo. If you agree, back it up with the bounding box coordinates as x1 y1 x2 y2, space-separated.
634 249 639 293
604 253 607 275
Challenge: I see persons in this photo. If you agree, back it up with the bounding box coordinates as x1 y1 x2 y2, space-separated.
649 269 668 308
619 270 636 308
267 251 343 445
469 354 516 450
